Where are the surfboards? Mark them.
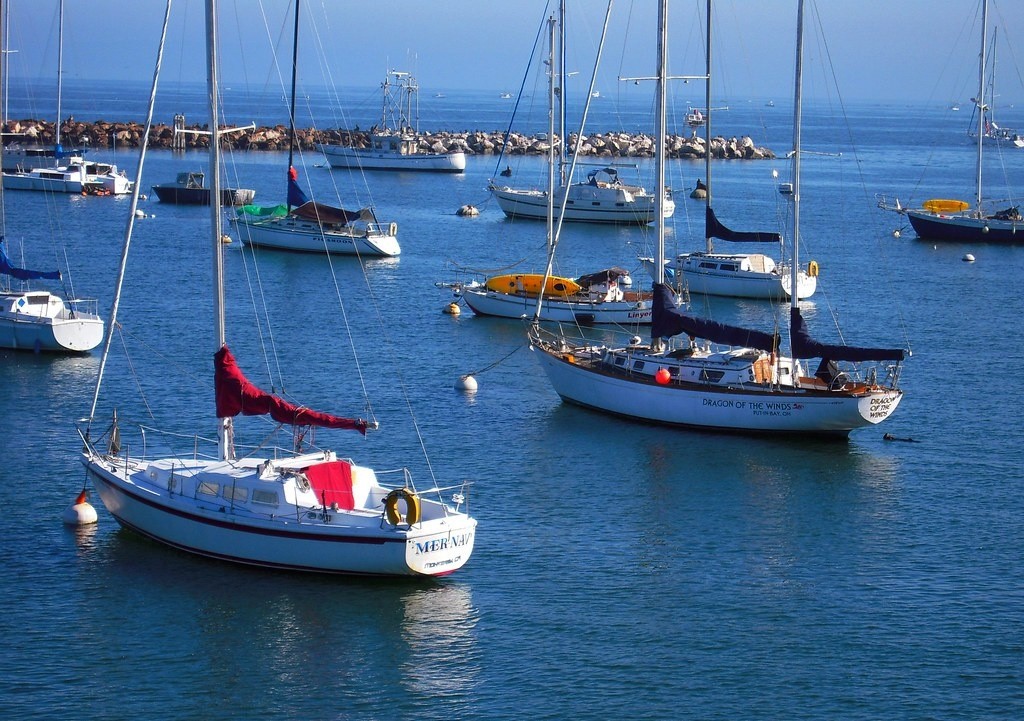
[922,200,969,213]
[486,274,581,298]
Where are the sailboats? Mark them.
[877,0,1024,244]
[524,0,916,433]
[486,0,677,226]
[636,0,821,300]
[433,0,692,326]
[0,0,107,355]
[0,0,135,196]
[964,22,1024,149]
[76,0,478,579]
[223,0,401,256]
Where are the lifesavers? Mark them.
[385,489,420,527]
[809,261,820,277]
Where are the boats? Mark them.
[592,91,601,99]
[764,100,776,107]
[951,106,959,111]
[434,93,447,99]
[779,180,793,194]
[312,50,469,174]
[150,171,258,204]
[684,109,706,126]
[498,92,513,100]
